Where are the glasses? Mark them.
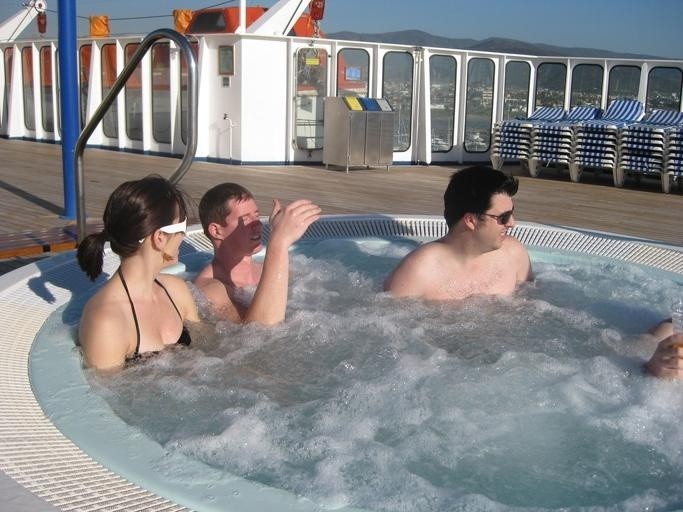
[476,206,515,226]
[159,216,188,236]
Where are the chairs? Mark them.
[491,98,683,195]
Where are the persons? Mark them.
[193,182,322,325]
[77,174,200,368]
[384,165,534,303]
[633,312,683,382]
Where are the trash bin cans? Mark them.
[322,97,397,174]
